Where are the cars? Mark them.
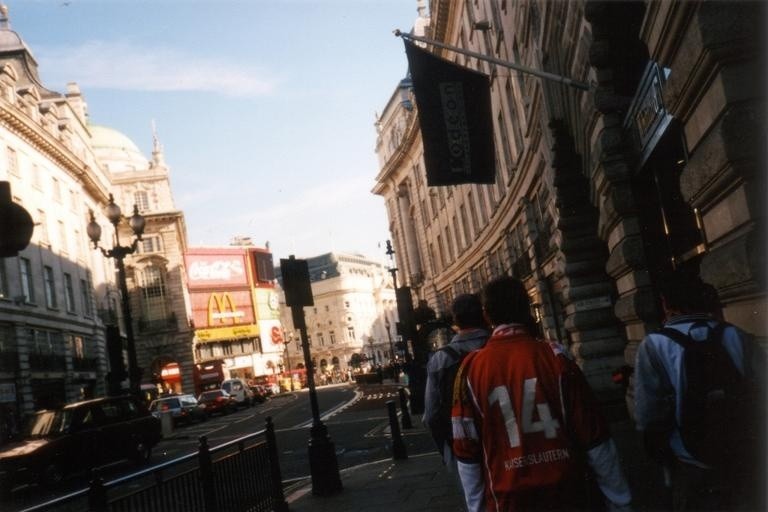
[148,373,280,426]
[1,397,162,487]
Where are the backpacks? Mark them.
[646,318,757,464]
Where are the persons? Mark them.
[632,271,766,512]
[420,292,493,495]
[448,275,636,512]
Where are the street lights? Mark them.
[86,194,145,391]
[367,335,378,365]
[383,309,394,358]
[282,326,293,391]
[385,240,399,290]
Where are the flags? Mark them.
[403,39,497,189]
